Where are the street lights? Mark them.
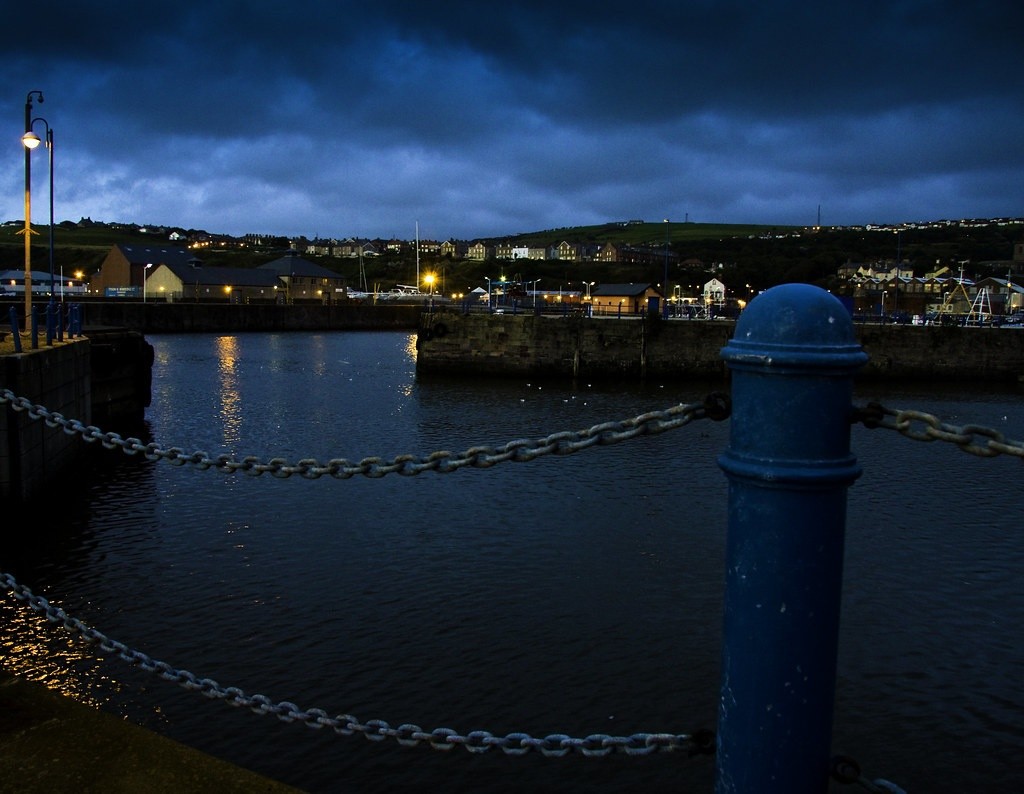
[745,283,751,306]
[582,281,590,298]
[588,282,596,313]
[25,89,46,333]
[143,263,153,302]
[426,274,435,295]
[484,276,492,311]
[23,116,55,306]
[662,218,671,297]
[532,278,542,308]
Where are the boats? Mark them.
[338,220,452,303]
